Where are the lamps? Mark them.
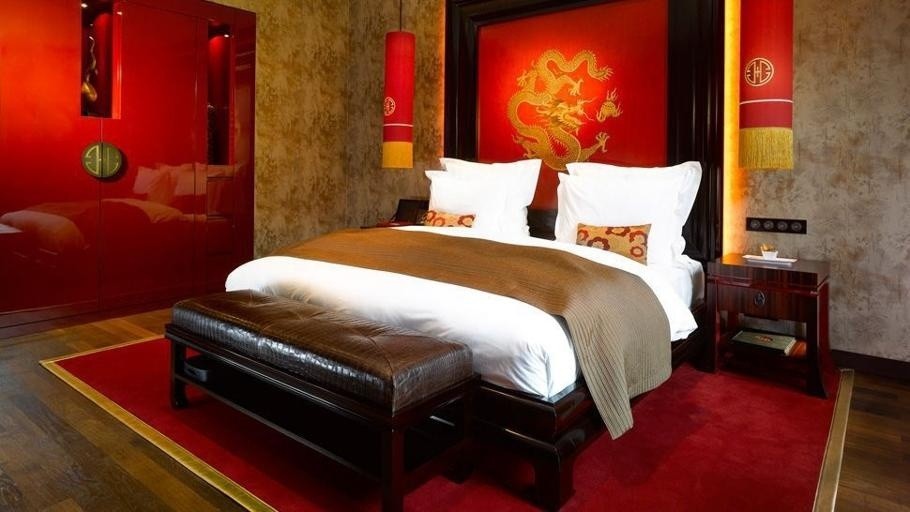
[738,1,796,173]
[379,0,417,172]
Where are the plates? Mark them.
[742,254,798,266]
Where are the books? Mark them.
[732,331,797,357]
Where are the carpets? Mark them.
[36,333,857,510]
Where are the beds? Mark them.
[224,198,705,510]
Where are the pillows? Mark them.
[420,157,704,266]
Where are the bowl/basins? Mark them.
[762,250,779,259]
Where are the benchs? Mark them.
[162,288,482,511]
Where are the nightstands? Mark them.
[702,254,833,398]
